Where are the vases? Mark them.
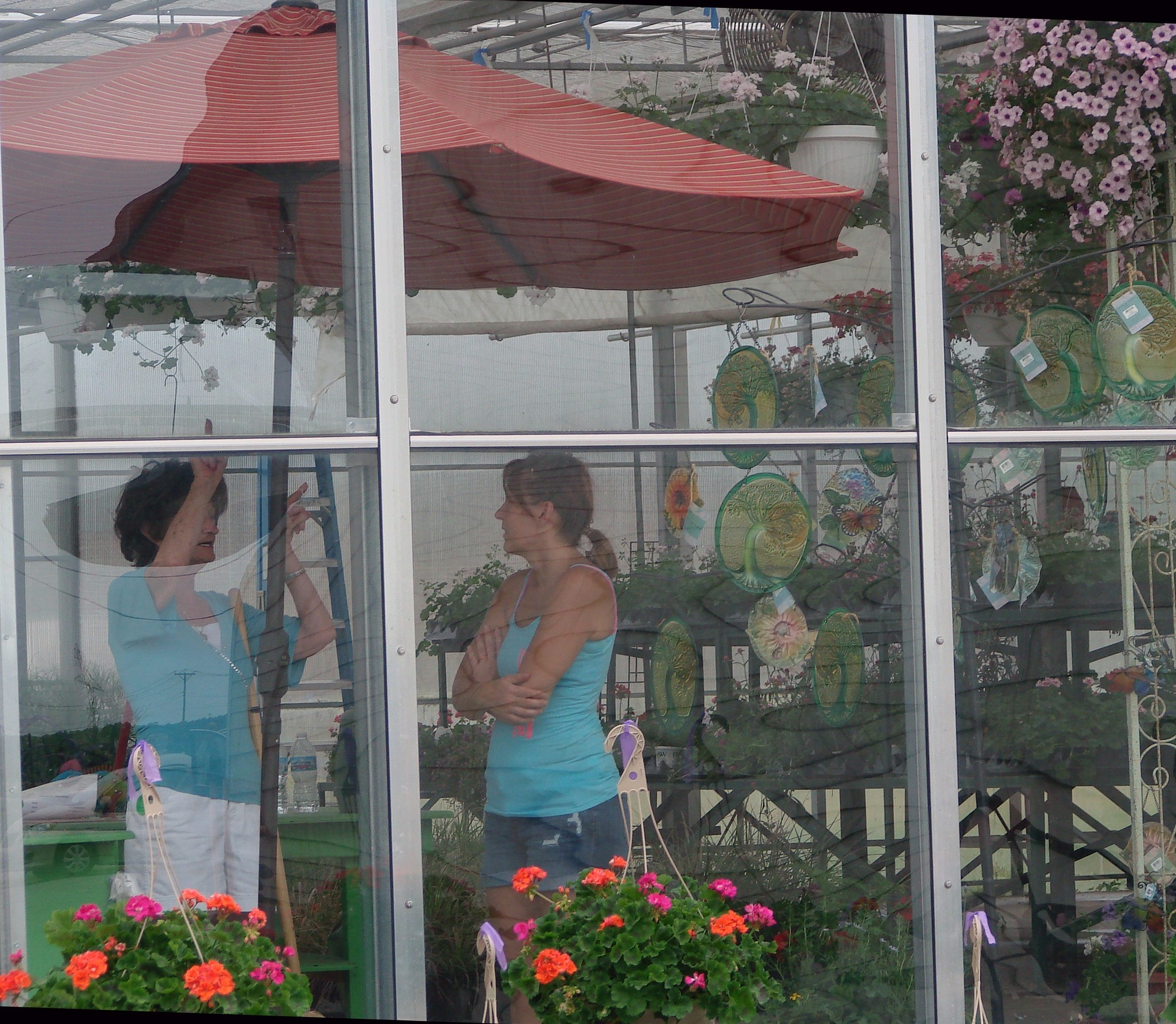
[30,287,110,345]
[961,303,1026,348]
[184,285,252,320]
[788,124,884,201]
[861,316,894,358]
[1125,969,1174,1020]
[108,283,176,333]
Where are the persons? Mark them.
[452,452,631,1022]
[104,454,337,910]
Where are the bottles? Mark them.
[291,732,320,813]
[277,744,288,813]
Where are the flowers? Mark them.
[281,843,500,1003]
[0,891,313,1018]
[572,19,1176,427]
[10,258,347,394]
[295,451,1176,1024]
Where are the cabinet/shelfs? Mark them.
[421,608,1176,995]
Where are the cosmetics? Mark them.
[0,0,868,947]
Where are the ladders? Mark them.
[256,452,354,937]
